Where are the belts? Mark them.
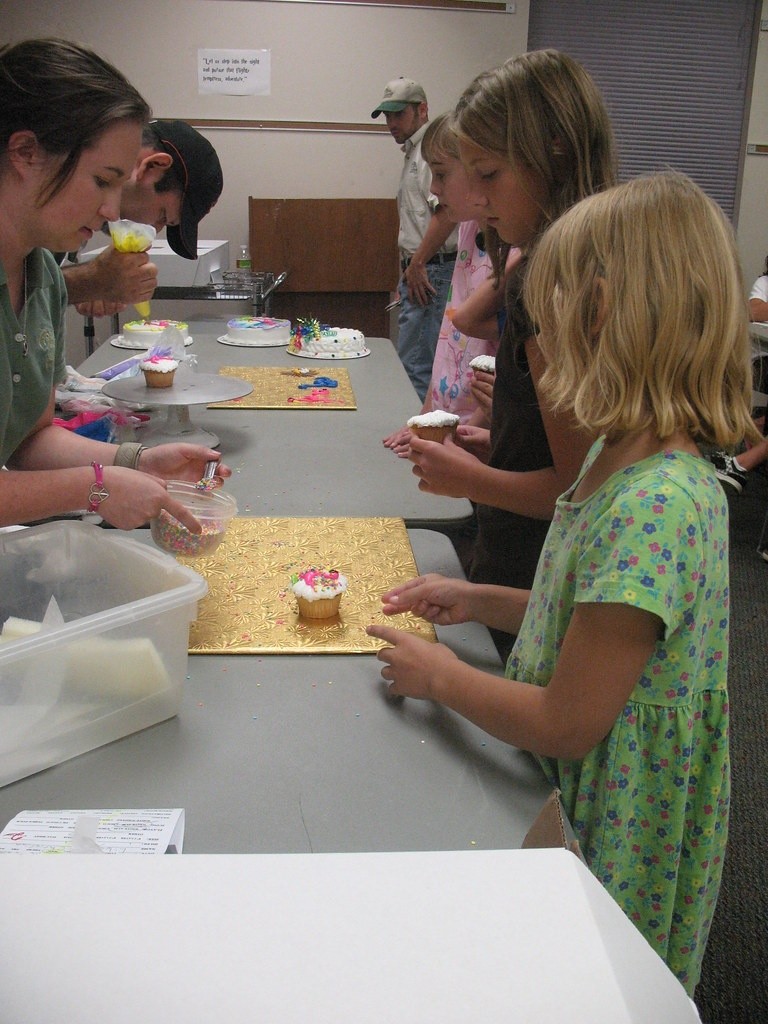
[401,252,459,272]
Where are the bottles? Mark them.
[235,245,253,290]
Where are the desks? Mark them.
[0,528,584,864]
[53,318,474,522]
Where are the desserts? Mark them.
[468,355,495,375]
[139,355,178,387]
[407,409,460,444]
[289,568,348,618]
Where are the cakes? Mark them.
[288,315,367,357]
[224,316,292,345]
[118,318,188,347]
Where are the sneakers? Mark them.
[702,449,748,496]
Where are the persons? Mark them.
[371,79,460,403]
[49,118,224,317]
[704,252,768,562]
[363,170,754,1003]
[409,47,617,662]
[0,36,233,533]
[381,107,526,463]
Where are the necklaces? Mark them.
[8,255,29,357]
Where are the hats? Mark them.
[149,118,222,260]
[371,77,426,119]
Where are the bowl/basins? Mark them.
[149,478,237,559]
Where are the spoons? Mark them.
[197,453,217,491]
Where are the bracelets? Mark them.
[87,460,109,513]
[113,441,148,470]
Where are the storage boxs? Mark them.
[0,521,206,792]
